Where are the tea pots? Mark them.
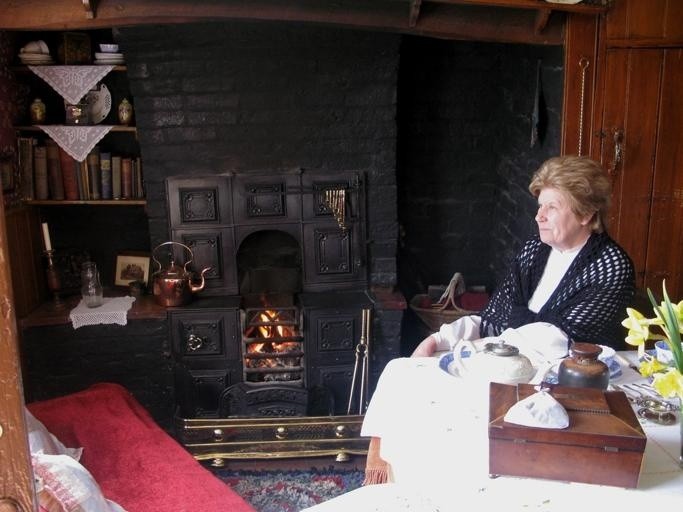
[148,241,212,305]
[453,338,554,389]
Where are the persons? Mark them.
[408,154,637,361]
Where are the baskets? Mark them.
[409,272,483,331]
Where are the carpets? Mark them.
[214,466,365,511]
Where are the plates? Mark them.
[63,84,111,125]
[438,349,467,378]
[637,348,657,376]
[92,53,124,65]
[18,53,51,64]
[604,359,621,375]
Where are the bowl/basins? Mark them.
[591,344,615,366]
[18,40,48,54]
[98,43,118,52]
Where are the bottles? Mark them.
[76,262,103,307]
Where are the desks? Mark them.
[359,345,683,510]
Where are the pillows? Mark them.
[21,405,129,512]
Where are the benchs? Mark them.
[16,382,256,512]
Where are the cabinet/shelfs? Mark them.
[561,0,683,349]
[1,30,168,325]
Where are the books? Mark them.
[16,138,146,201]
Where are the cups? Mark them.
[653,340,674,364]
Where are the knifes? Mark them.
[613,382,678,406]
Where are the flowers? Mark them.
[622,277,683,414]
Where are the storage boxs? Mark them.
[488,381,647,490]
